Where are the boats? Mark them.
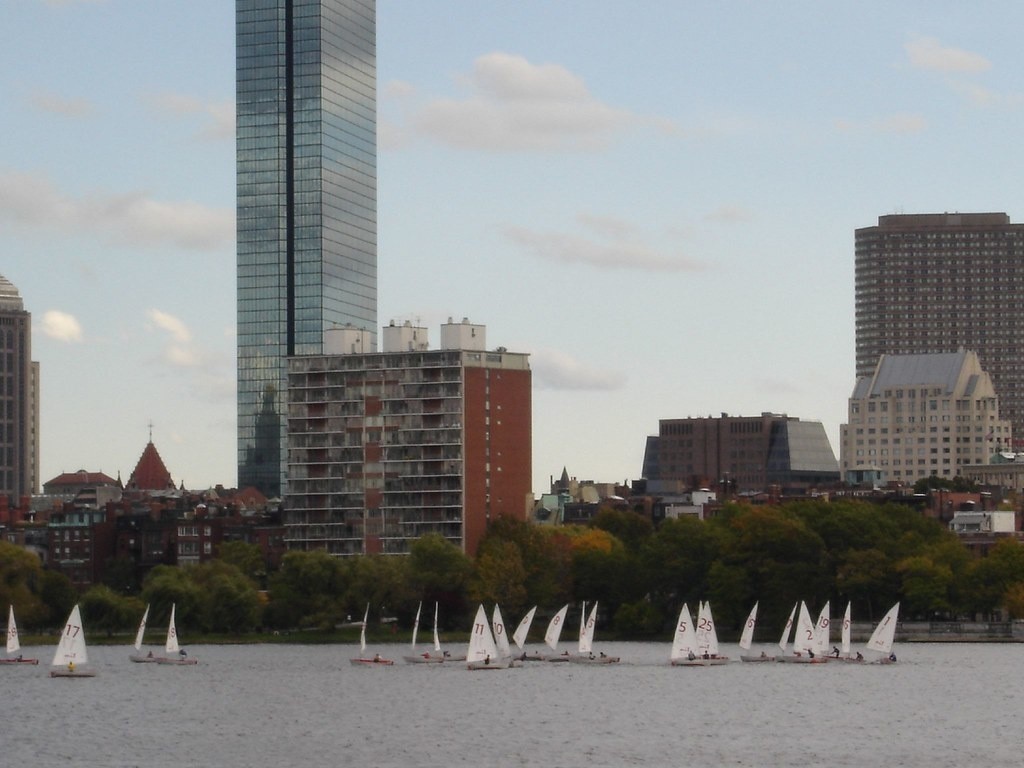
[0,605,39,666]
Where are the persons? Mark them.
[421,650,431,660]
[889,652,896,661]
[808,650,814,658]
[857,652,863,661]
[446,651,450,657]
[519,652,527,661]
[373,653,380,663]
[565,650,569,655]
[68,662,74,672]
[703,650,709,659]
[688,650,696,660]
[600,652,606,658]
[485,655,490,665]
[589,651,594,660]
[761,652,766,658]
[831,647,839,657]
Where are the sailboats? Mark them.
[466,603,523,670]
[544,601,621,667]
[350,602,394,666]
[739,600,900,664]
[513,605,545,661]
[670,600,729,666]
[130,604,198,664]
[403,601,467,663]
[48,605,96,678]
